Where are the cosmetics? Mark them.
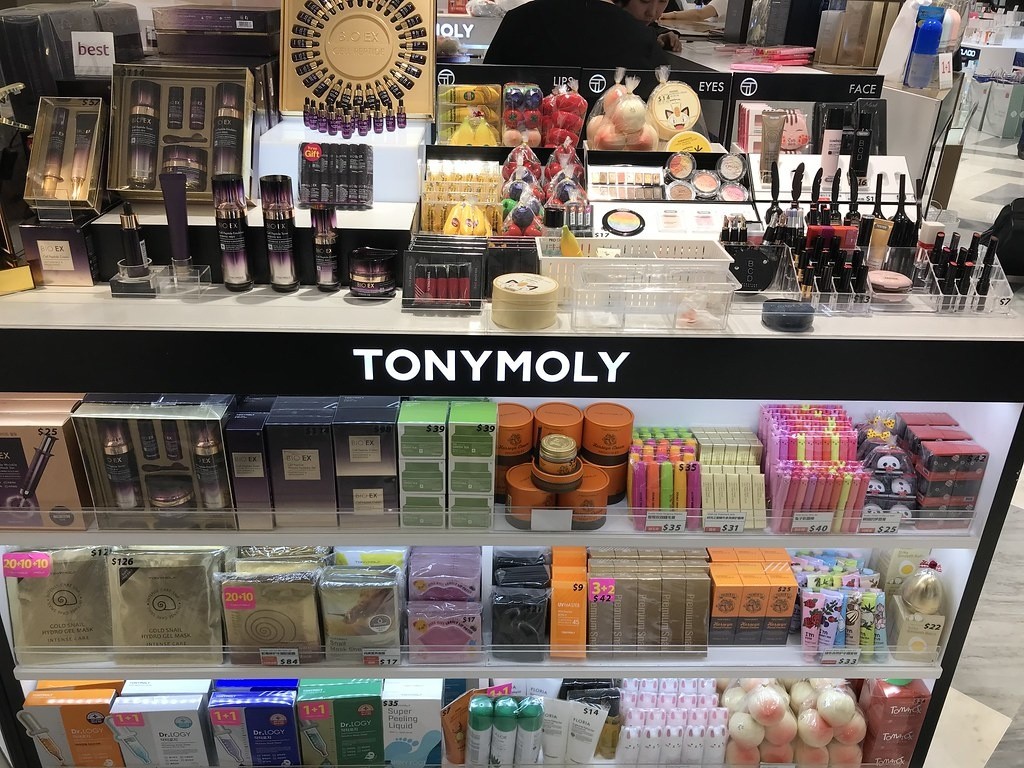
[0,0,999,768]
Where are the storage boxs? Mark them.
[0,391,798,768]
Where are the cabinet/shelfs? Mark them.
[659,41,835,155]
[0,281,1024,768]
[876,70,965,223]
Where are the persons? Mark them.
[656,0,728,22]
[618,0,682,54]
